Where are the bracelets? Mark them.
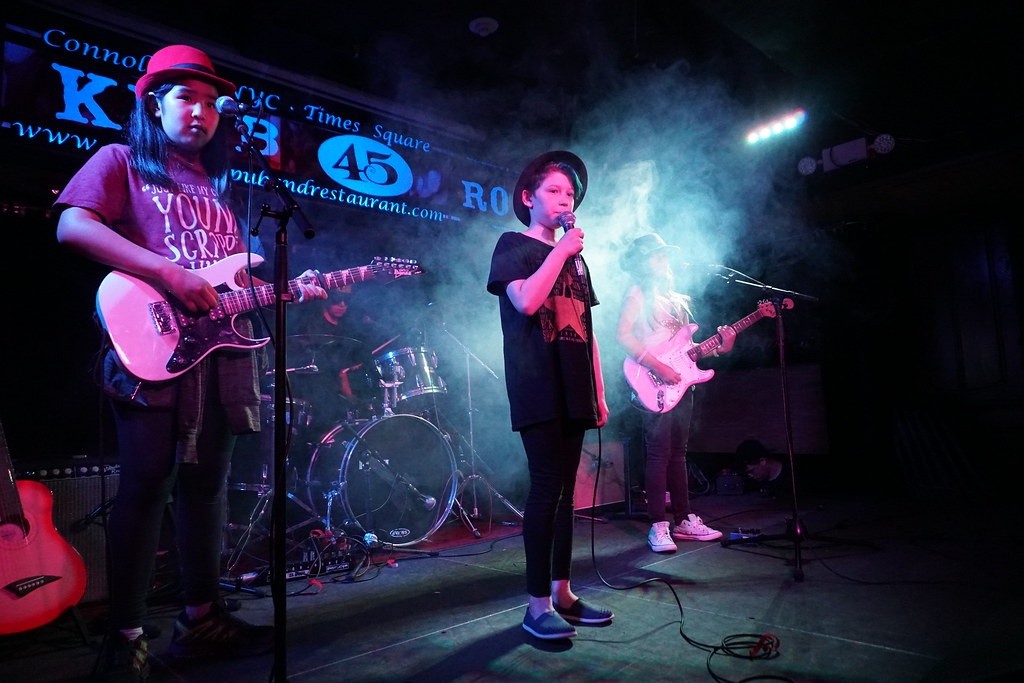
[635,350,649,364]
[712,349,720,357]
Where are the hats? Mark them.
[135,45,237,101]
[619,233,680,270]
[513,151,588,228]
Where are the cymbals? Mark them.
[287,334,372,368]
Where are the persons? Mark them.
[322,279,353,325]
[49,42,330,683]
[733,438,798,498]
[612,234,737,552]
[484,149,614,640]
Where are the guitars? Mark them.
[622,297,784,415]
[0,425,89,636]
[95,252,429,386]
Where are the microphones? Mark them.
[683,263,724,271]
[558,211,585,284]
[85,501,114,523]
[216,95,249,117]
[417,494,437,511]
[591,462,614,470]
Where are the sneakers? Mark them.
[522,607,575,639]
[553,598,612,623]
[94,623,163,683]
[673,514,723,541]
[168,597,276,666]
[648,520,677,552]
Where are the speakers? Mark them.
[10,455,120,604]
[572,433,628,516]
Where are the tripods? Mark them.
[224,345,440,583]
[711,270,874,583]
[455,381,524,520]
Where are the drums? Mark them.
[251,396,309,465]
[305,412,461,550]
[367,346,449,412]
